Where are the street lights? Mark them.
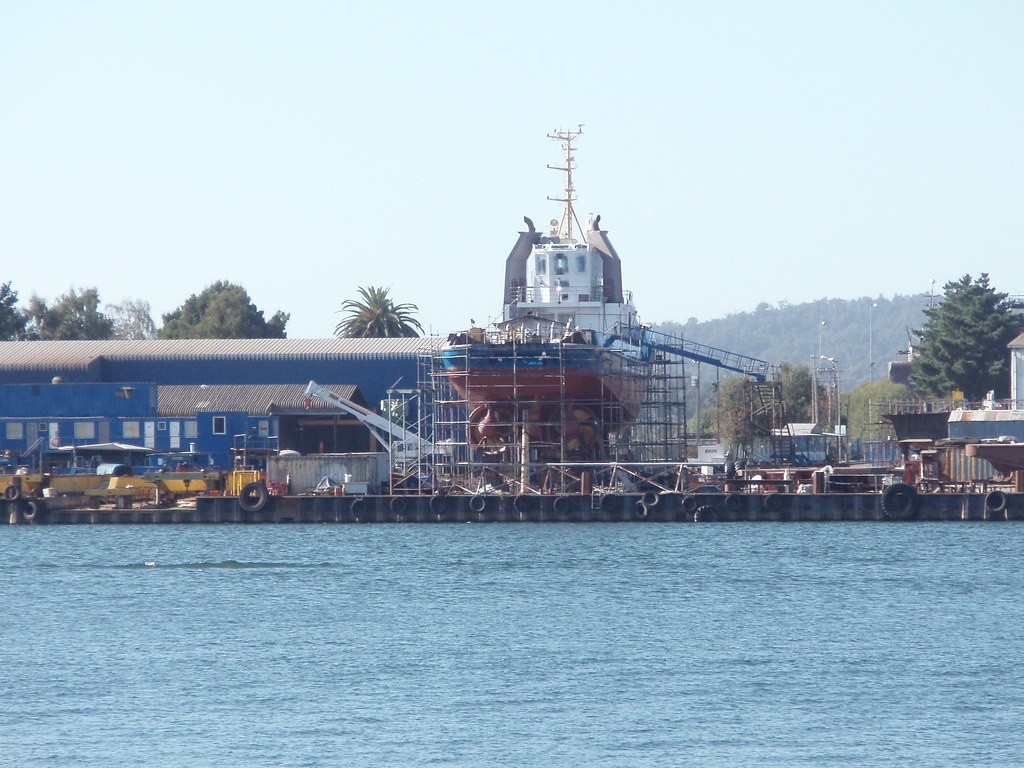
[819,355,841,460]
[818,319,827,369]
[868,302,878,459]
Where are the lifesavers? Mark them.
[555,497,572,514]
[513,494,535,511]
[763,494,785,513]
[725,493,741,511]
[389,496,407,515]
[469,496,487,512]
[985,489,1009,514]
[22,499,40,521]
[681,495,698,512]
[599,493,618,512]
[3,484,19,502]
[644,491,660,508]
[634,500,649,519]
[349,498,367,519]
[429,496,449,514]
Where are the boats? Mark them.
[438,121,772,490]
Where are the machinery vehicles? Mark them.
[302,380,455,495]
